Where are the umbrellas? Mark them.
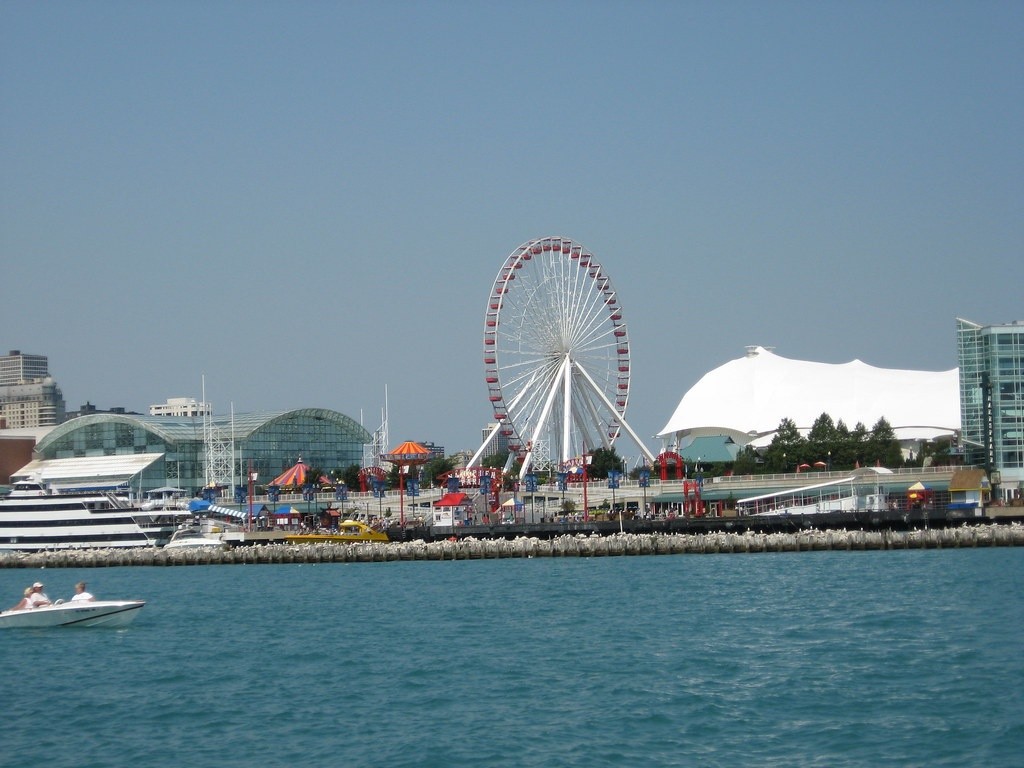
[502,498,524,521]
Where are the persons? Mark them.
[71,582,95,602]
[296,516,423,538]
[455,492,1021,523]
[11,582,54,611]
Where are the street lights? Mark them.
[909,445,912,465]
[828,450,833,470]
[783,453,786,473]
[621,455,626,480]
[698,457,702,474]
[625,461,628,479]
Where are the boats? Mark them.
[0,598,147,632]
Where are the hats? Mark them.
[33,583,43,589]
